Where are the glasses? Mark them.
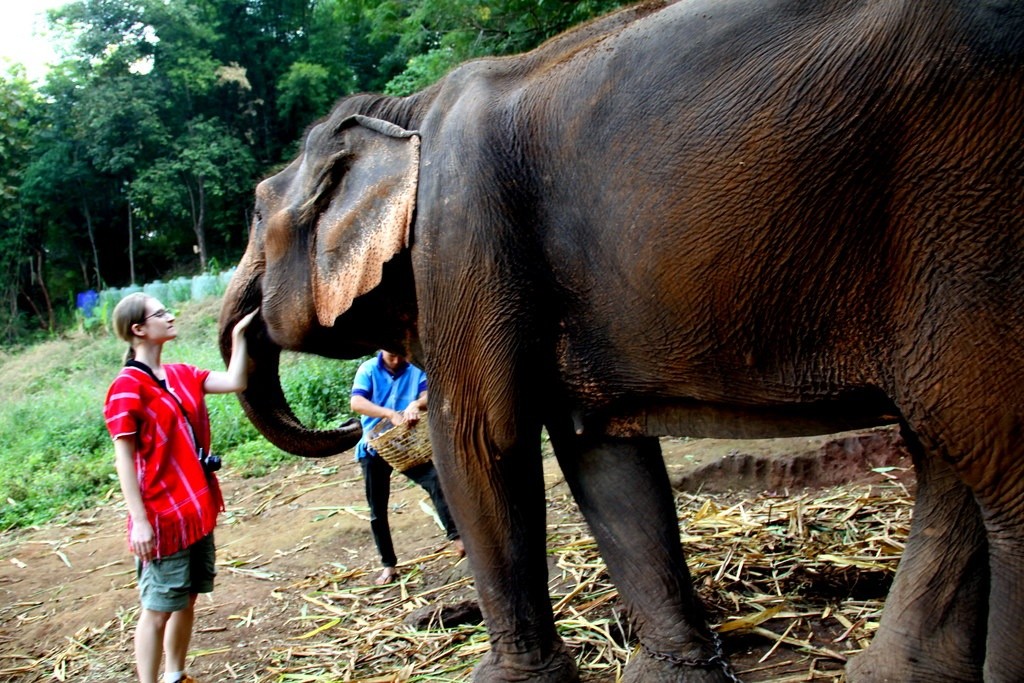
[144,308,171,320]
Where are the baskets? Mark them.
[366,410,431,472]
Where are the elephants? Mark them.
[217,0,1024,682]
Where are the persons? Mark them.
[350,349,468,589]
[101,291,260,683]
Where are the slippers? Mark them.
[381,572,399,585]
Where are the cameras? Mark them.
[197,448,222,480]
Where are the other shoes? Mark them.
[173,674,202,683]
